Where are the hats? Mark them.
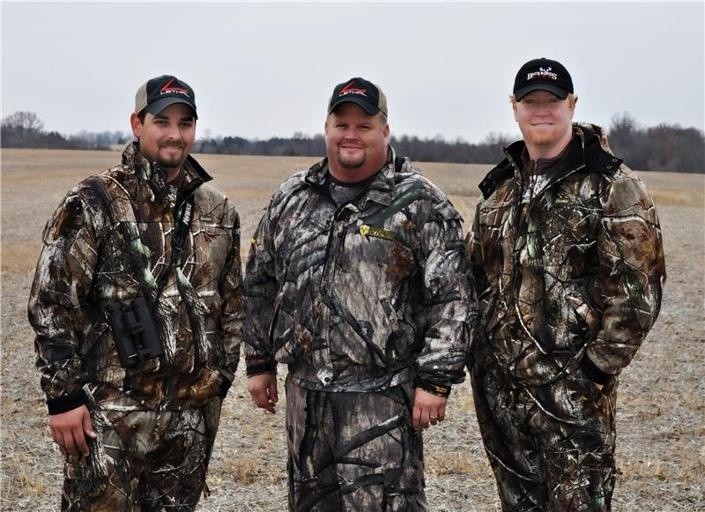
[512,58,574,102]
[134,72,198,120]
[327,76,388,118]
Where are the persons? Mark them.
[27,74,247,511]
[461,57,668,512]
[243,76,481,511]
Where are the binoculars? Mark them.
[107,297,163,368]
